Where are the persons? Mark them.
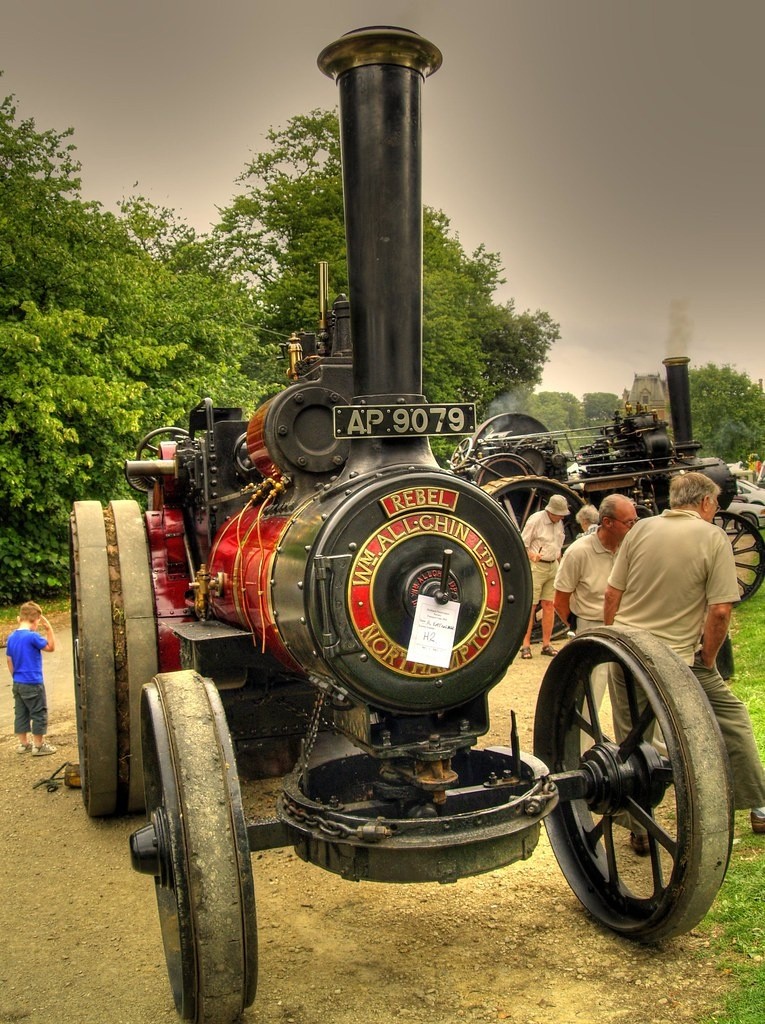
[566,451,590,491]
[6,601,57,756]
[520,493,668,757]
[604,472,765,855]
[747,453,765,484]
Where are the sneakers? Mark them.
[32,744,57,755]
[18,742,33,753]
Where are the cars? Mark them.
[728,463,765,505]
[712,502,764,533]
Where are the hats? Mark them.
[545,495,571,515]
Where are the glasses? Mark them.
[601,515,641,528]
[713,506,721,513]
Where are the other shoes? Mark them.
[750,812,765,833]
[630,831,658,857]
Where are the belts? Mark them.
[539,559,556,563]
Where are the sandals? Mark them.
[520,648,533,659]
[541,644,559,656]
